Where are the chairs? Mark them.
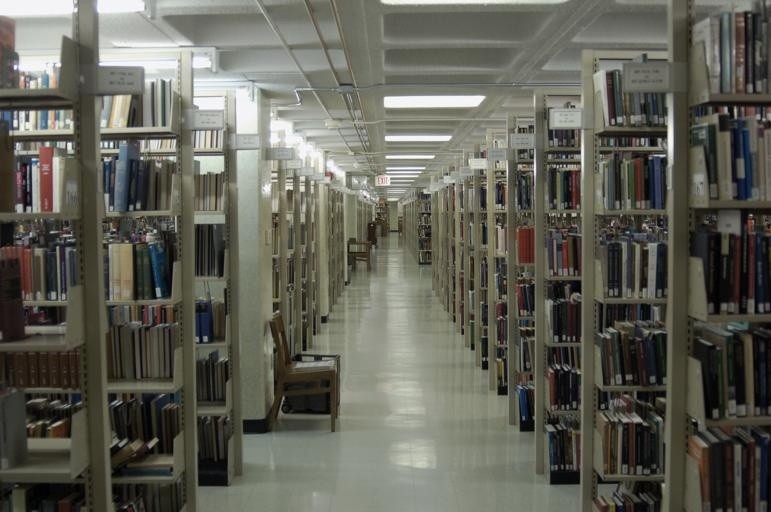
[265,308,343,435]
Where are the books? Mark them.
[686,1,770,511]
[515,125,534,422]
[271,182,317,356]
[544,101,582,471]
[98,78,187,510]
[1,16,85,511]
[593,52,666,511]
[193,130,229,464]
[494,140,508,386]
[419,191,431,262]
[446,150,488,369]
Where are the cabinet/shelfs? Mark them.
[403,0,771,511]
[1,0,373,512]
